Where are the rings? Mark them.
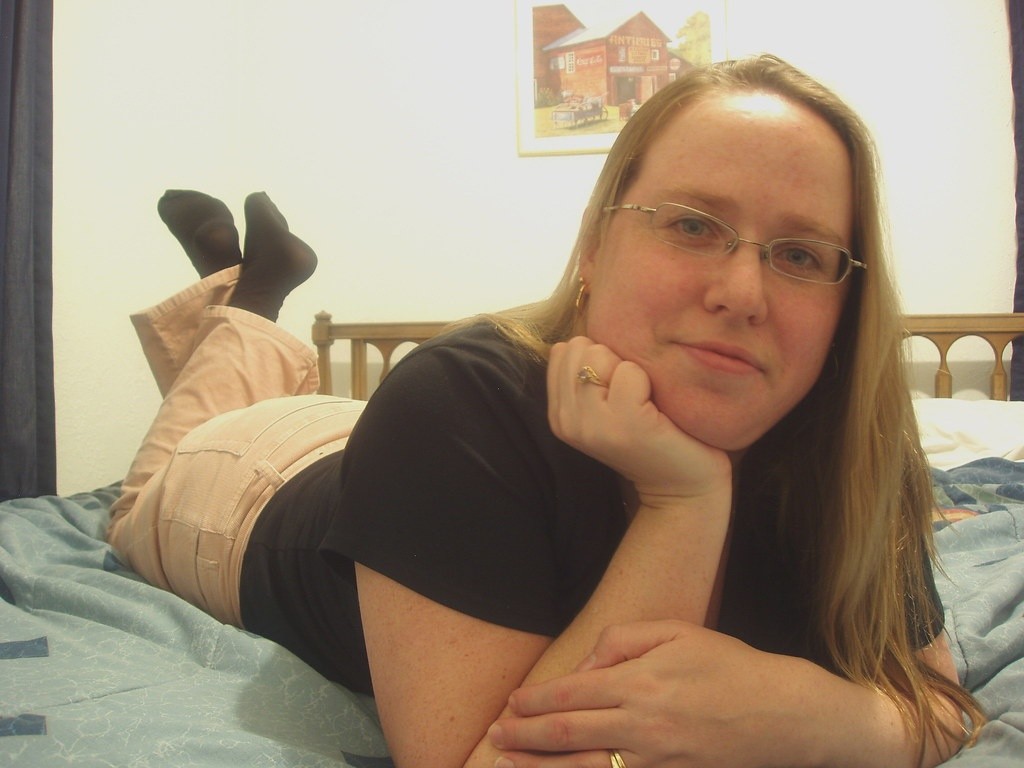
[609,750,626,768]
[575,365,611,388]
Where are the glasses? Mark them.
[602,202,868,286]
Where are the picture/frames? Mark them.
[513,0,730,158]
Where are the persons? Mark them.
[102,60,983,768]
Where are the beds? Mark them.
[0,312,1024,767]
